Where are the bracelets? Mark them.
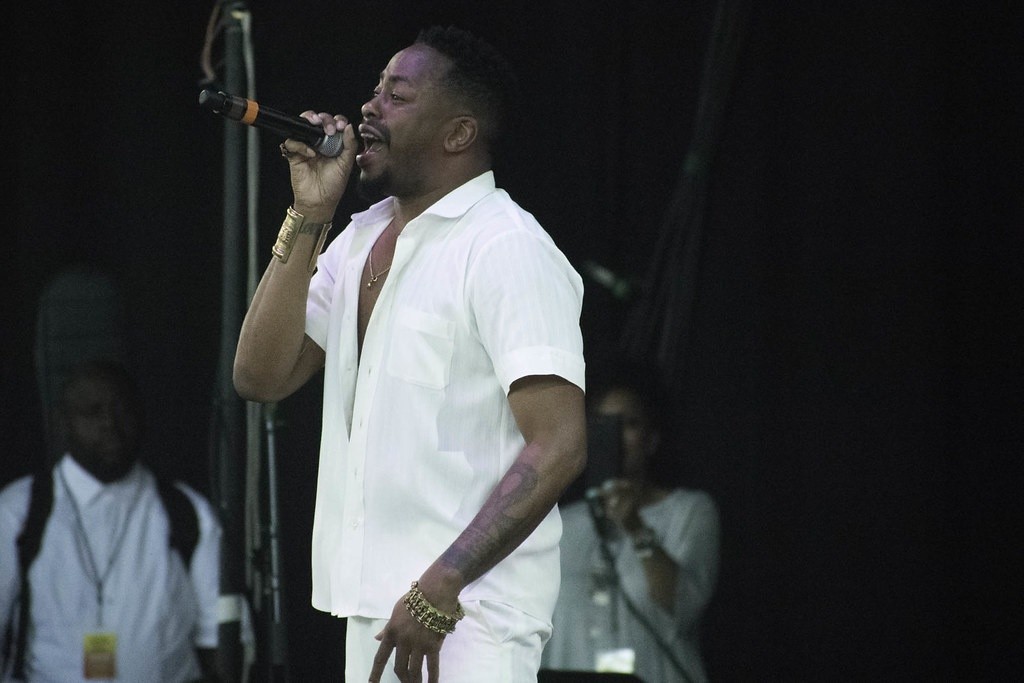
[271,205,332,273]
[405,582,466,634]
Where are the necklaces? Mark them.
[367,252,391,289]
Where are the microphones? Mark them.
[586,485,609,537]
[199,90,344,158]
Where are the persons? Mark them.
[231,27,586,682]
[0,359,206,683]
[541,382,722,683]
